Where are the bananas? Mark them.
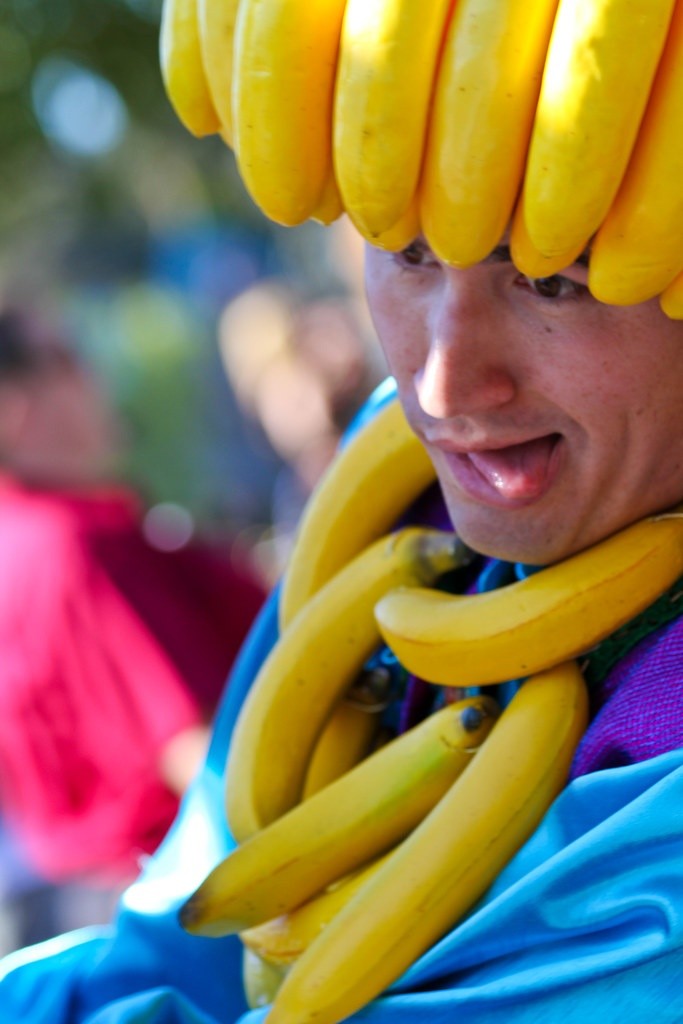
[178,396,683,1024]
[160,0,683,323]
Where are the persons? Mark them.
[2,276,276,939]
[223,285,385,535]
[0,1,682,1024]
